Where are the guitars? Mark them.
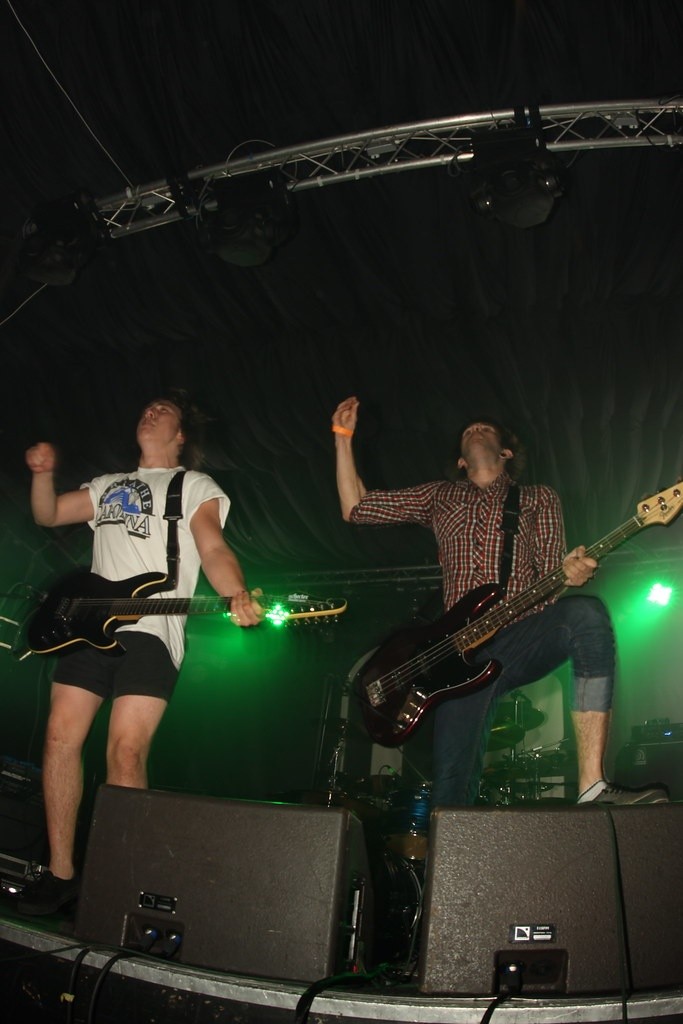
[21,568,348,658]
[356,473,683,749]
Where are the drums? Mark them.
[367,849,424,971]
[378,786,432,860]
[496,754,556,799]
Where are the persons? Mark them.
[330,393,670,807]
[17,387,266,916]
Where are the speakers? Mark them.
[73,783,375,984]
[418,806,683,996]
[623,740,683,803]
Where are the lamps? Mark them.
[12,188,112,287]
[469,125,564,229]
[194,179,297,267]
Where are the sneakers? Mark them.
[402,960,419,977]
[17,870,80,915]
[593,784,669,804]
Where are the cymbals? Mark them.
[265,788,383,824]
[485,719,526,753]
[494,701,546,731]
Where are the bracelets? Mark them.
[332,424,353,437]
